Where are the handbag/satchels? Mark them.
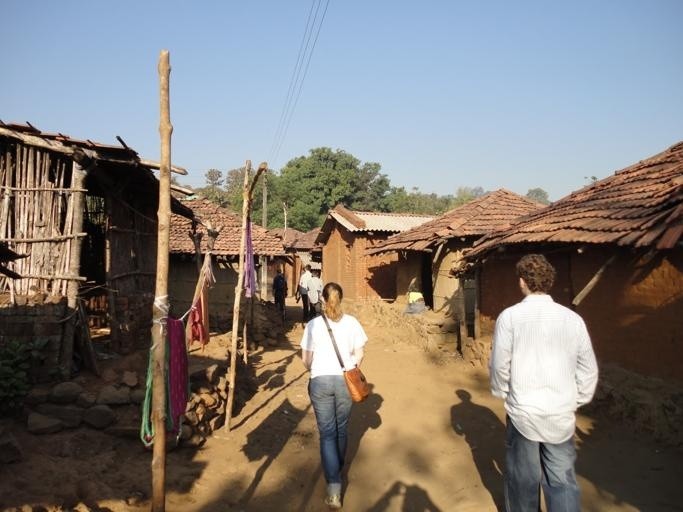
[342,367,370,403]
[306,289,320,305]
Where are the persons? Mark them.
[299,281,368,507]
[295,264,313,324]
[294,266,306,300]
[488,253,600,512]
[271,268,287,320]
[306,268,326,321]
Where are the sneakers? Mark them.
[324,496,342,509]
